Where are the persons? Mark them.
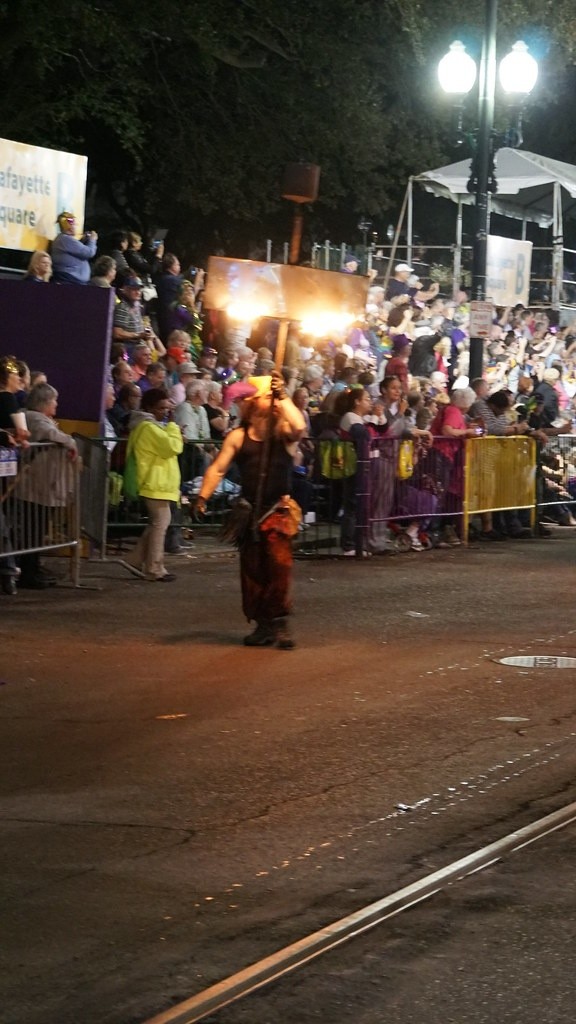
[23,211,576,557]
[117,388,183,582]
[0,353,78,586]
[188,369,306,649]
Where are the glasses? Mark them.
[58,215,74,226]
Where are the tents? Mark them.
[407,146,576,301]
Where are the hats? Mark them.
[124,275,144,287]
[201,347,219,355]
[177,362,201,374]
[391,334,413,348]
[395,264,415,272]
[344,254,361,263]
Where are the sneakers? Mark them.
[401,529,426,552]
[428,530,448,547]
[443,525,461,545]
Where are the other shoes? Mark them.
[364,538,406,554]
[340,542,371,557]
[273,620,297,650]
[244,622,274,646]
[480,528,506,541]
[558,512,576,526]
[290,546,316,560]
[121,559,143,577]
[164,547,188,556]
[165,533,196,549]
[16,567,57,585]
[143,572,178,582]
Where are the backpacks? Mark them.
[123,423,156,502]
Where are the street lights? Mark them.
[437,39,538,388]
